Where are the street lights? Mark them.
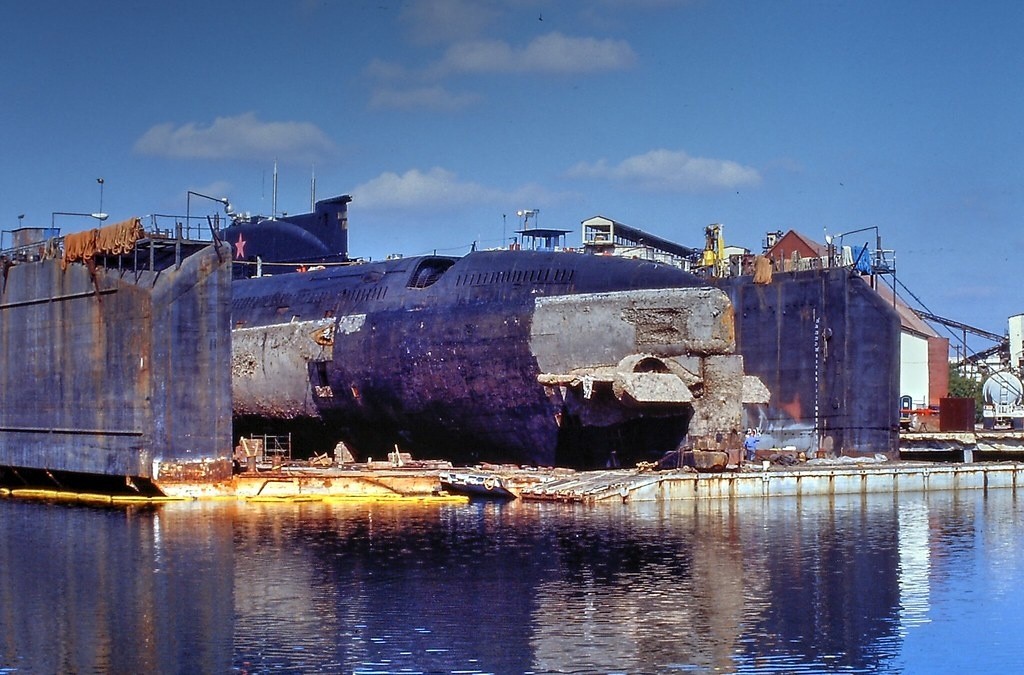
[185,190,234,241]
[823,226,880,273]
[52,211,108,229]
[17,214,25,228]
[95,178,105,231]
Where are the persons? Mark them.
[745,433,760,461]
